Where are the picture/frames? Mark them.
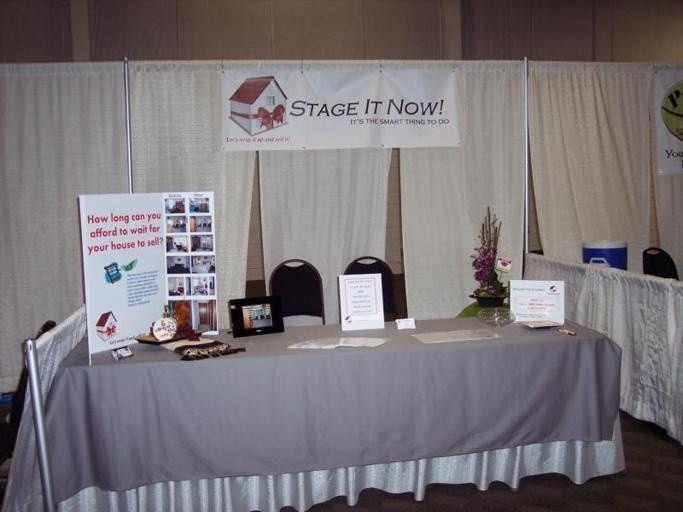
[470,295,509,307]
[229,296,285,338]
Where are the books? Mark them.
[286,334,391,349]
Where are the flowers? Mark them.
[470,206,511,297]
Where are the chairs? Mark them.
[643,246,680,281]
[343,255,396,321]
[269,259,326,326]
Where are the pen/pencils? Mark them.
[557,328,576,335]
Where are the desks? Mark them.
[57,311,606,512]
